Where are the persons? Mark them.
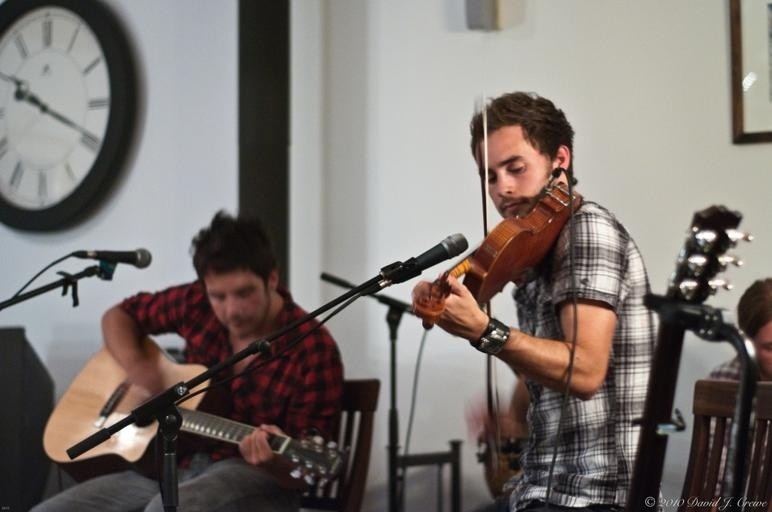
[701,278,772,511]
[464,373,532,512]
[27,208,342,511]
[435,90,661,512]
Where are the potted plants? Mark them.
[729,0,772,144]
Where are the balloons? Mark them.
[0,0,139,233]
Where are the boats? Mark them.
[466,0,527,31]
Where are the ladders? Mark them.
[414,181,583,330]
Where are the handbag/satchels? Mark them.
[470,317,510,356]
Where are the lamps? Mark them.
[676,378,772,512]
[299,379,380,512]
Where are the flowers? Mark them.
[72,248,153,269]
[360,232,469,297]
[515,168,562,219]
